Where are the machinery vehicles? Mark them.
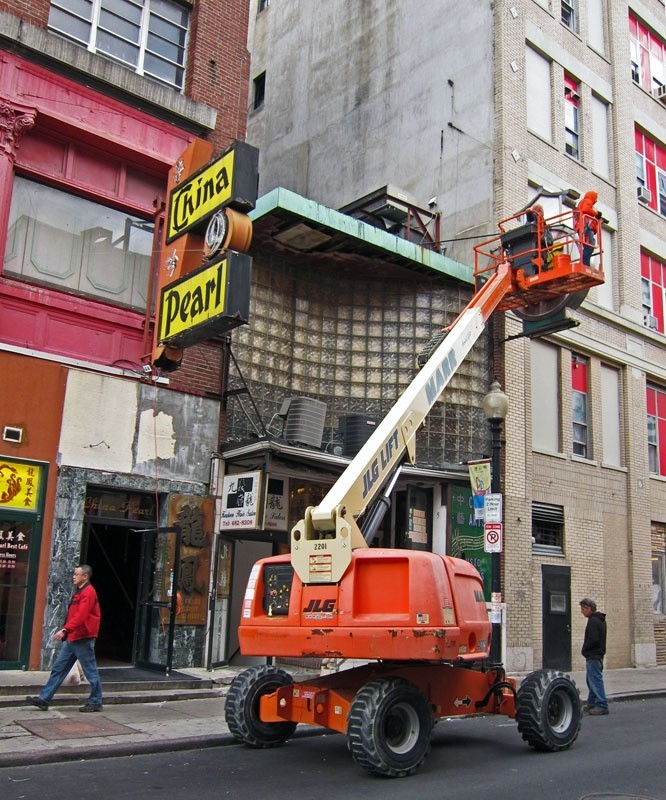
[222,190,607,779]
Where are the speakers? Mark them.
[339,414,381,460]
[279,396,327,449]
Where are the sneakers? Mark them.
[79,704,103,712]
[26,696,48,711]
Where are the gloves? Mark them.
[601,218,609,224]
[597,212,602,219]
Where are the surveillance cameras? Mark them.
[427,196,438,209]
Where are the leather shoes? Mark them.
[583,706,593,712]
[589,708,609,715]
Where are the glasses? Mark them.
[73,573,83,576]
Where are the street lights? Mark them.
[479,381,507,670]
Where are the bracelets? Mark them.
[62,629,67,634]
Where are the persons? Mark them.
[527,205,552,271]
[579,598,610,715]
[576,191,602,266]
[28,563,104,712]
